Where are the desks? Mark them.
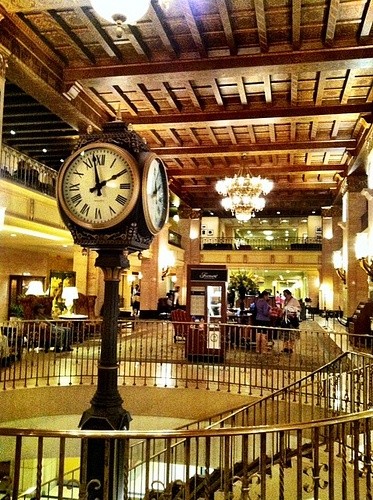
[59,315,88,344]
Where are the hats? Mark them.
[34,304,43,309]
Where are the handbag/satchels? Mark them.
[251,302,257,317]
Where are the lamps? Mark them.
[333,251,346,284]
[355,233,373,282]
[214,155,274,221]
[89,0,151,38]
[61,287,79,316]
[25,281,43,295]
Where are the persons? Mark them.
[33,303,74,352]
[172,285,181,310]
[254,290,273,355]
[131,284,141,319]
[276,290,302,353]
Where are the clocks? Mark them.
[58,141,141,231]
[141,152,169,235]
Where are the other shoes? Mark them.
[281,348,293,354]
[53,348,61,353]
[62,347,73,351]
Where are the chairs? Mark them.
[19,294,64,351]
[171,309,187,343]
[74,293,135,337]
